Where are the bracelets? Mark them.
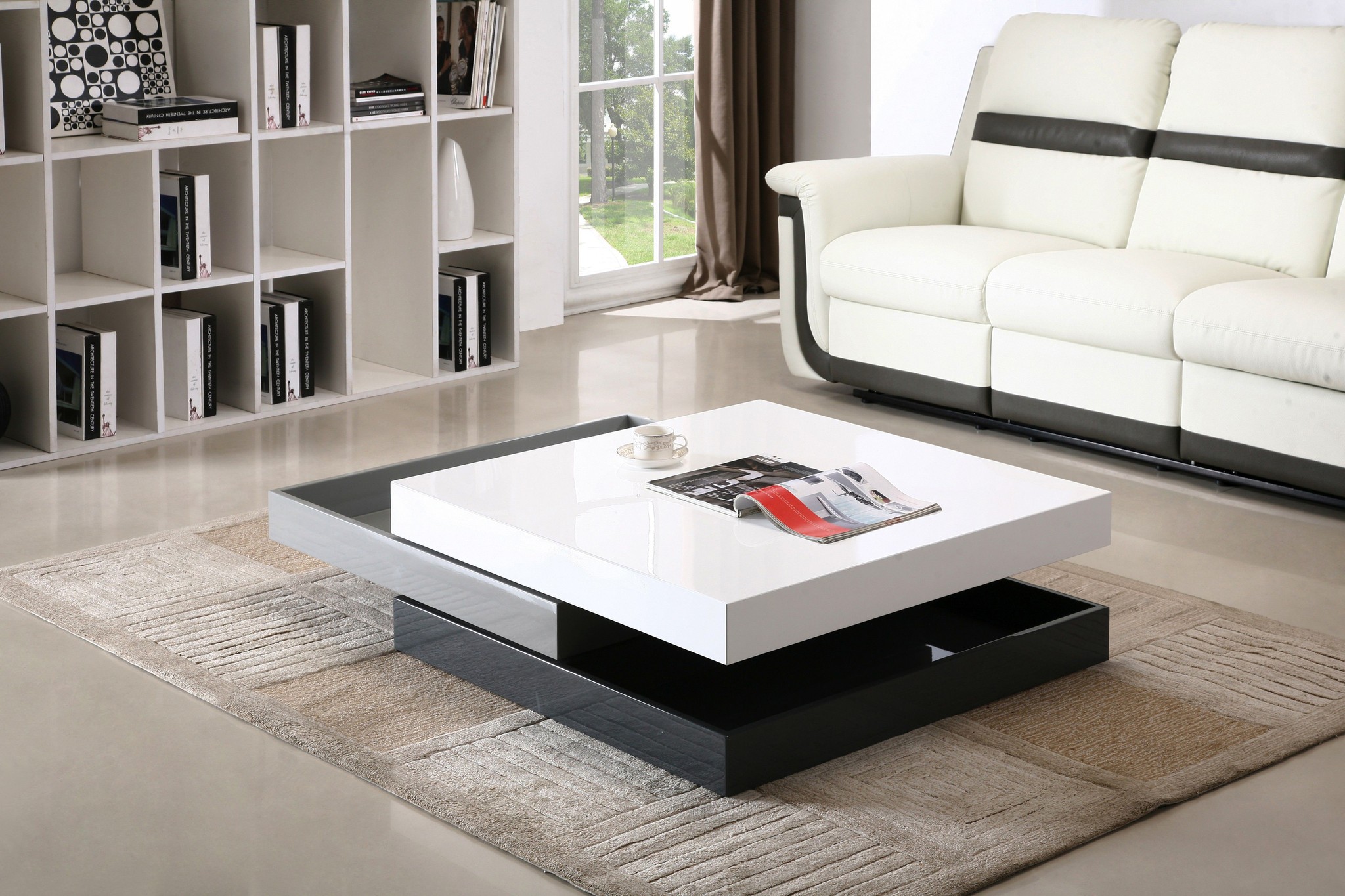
[438,72,442,76]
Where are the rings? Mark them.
[450,59,451,60]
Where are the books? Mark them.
[261,290,315,405]
[438,266,491,372]
[350,73,426,122]
[159,171,212,281]
[56,321,116,441]
[646,454,942,544]
[436,0,506,109]
[101,91,239,142]
[256,22,310,129]
[162,307,217,421]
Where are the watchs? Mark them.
[449,58,468,95]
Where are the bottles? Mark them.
[438,136,474,241]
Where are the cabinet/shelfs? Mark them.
[0,0,523,469]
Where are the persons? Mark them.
[437,16,452,95]
[457,5,477,96]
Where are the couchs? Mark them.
[764,14,1345,513]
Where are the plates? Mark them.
[616,442,689,469]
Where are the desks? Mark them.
[263,405,1115,799]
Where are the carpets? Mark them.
[0,499,1344,896]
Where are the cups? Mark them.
[633,426,688,460]
